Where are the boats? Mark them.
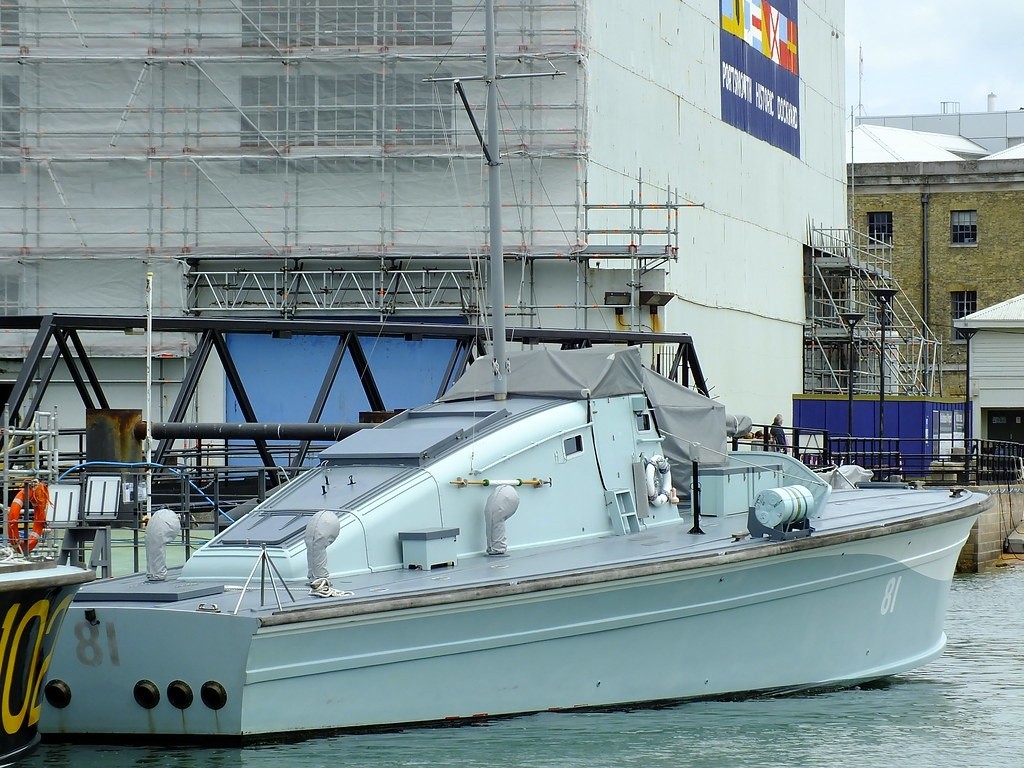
[0,390,97,768]
[38,0,997,750]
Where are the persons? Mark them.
[770,413,788,454]
[741,431,764,439]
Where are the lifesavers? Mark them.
[7,487,46,554]
